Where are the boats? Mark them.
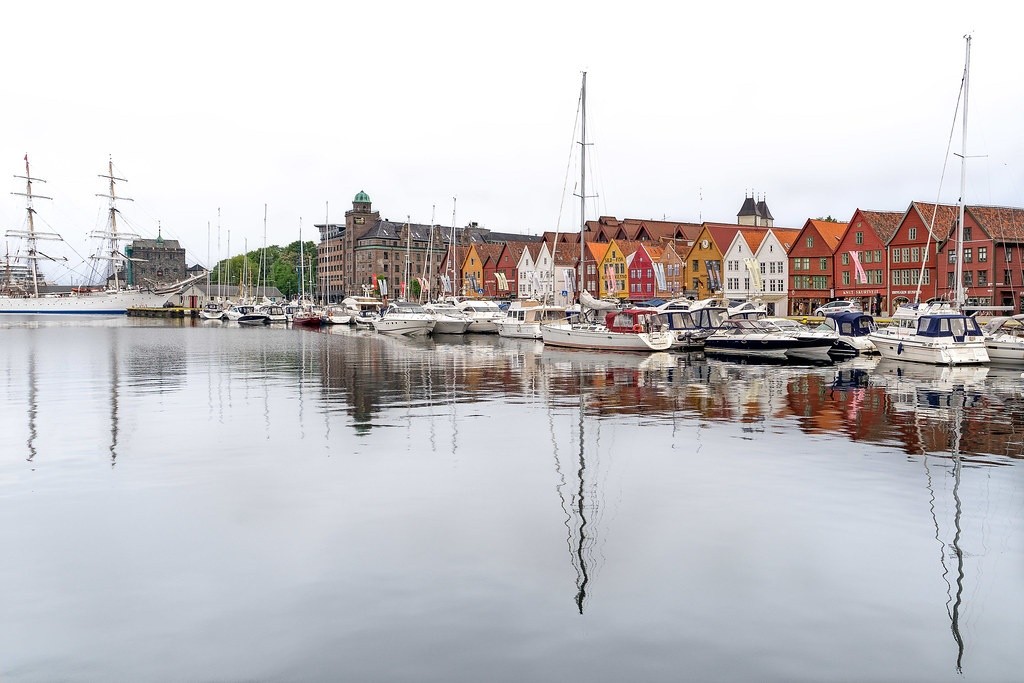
[199,31,879,360]
[983,313,1024,367]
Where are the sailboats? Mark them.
[538,70,675,352]
[866,32,990,365]
[0,150,213,317]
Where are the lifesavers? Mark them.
[633,324,642,332]
[328,312,332,316]
[578,313,587,323]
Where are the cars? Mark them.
[813,300,864,318]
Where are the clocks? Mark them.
[702,240,708,249]
